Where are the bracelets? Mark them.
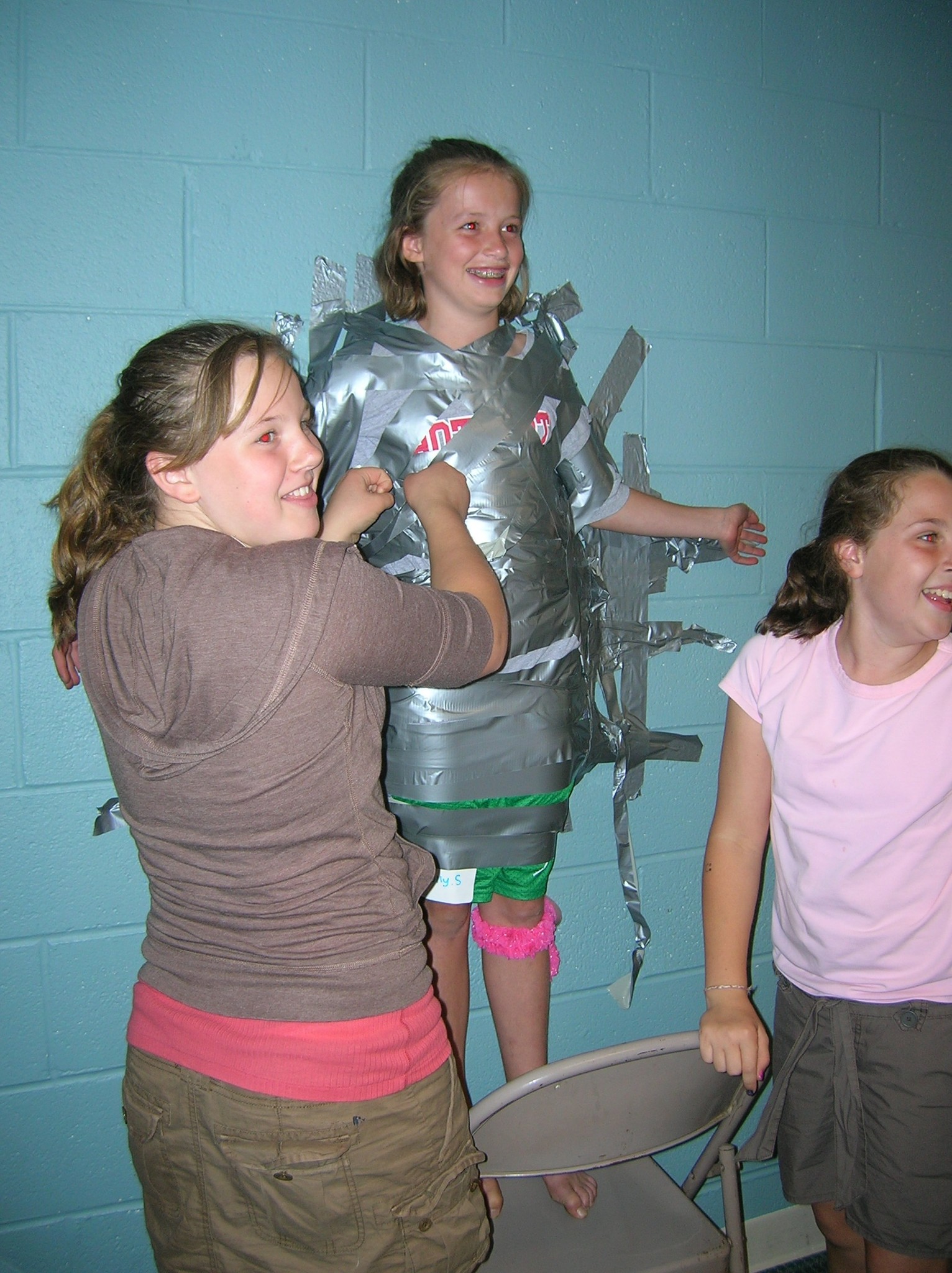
[704,983,754,997]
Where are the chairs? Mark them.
[467,1030,767,1272]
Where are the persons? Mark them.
[46,135,769,1226]
[40,321,504,1273]
[699,449,951,1273]
